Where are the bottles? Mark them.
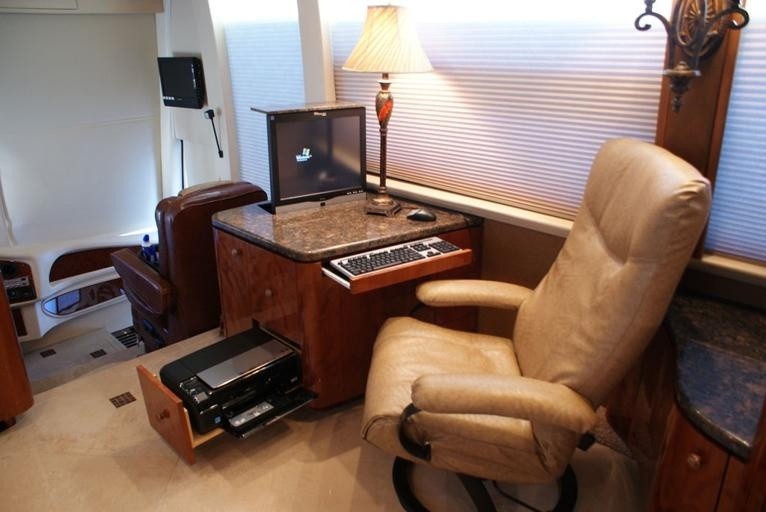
[142,235,157,262]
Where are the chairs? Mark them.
[359,136,712,512]
[109,180,267,354]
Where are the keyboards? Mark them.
[329,235,462,280]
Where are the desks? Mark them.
[135,192,483,466]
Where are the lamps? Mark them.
[341,4,435,218]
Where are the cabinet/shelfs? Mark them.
[605,323,766,512]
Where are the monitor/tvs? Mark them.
[257,105,367,215]
[157,57,205,109]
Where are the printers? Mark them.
[160,328,319,438]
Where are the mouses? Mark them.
[407,209,436,221]
